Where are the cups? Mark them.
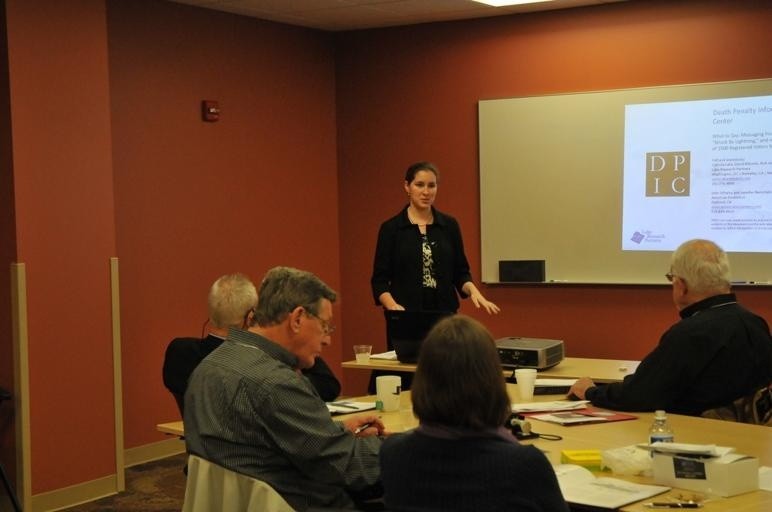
[353,344,373,362]
[516,368,537,400]
[376,374,401,412]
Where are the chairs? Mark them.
[181,454,294,512]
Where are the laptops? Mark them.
[384,310,456,363]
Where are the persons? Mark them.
[370,160,501,364]
[565,239,771,427]
[182,263,392,512]
[160,271,343,474]
[379,312,570,512]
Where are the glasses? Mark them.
[296,304,336,336]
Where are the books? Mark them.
[554,463,672,511]
[520,413,639,427]
[327,401,378,417]
[511,400,592,412]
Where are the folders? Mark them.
[516,404,639,425]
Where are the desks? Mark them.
[159,380,772,512]
[342,348,642,384]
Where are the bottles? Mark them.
[649,410,673,457]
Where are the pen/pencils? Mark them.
[331,403,359,409]
[354,416,382,434]
[643,501,704,509]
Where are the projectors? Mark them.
[495,336,564,369]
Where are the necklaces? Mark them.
[410,210,433,227]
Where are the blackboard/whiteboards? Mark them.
[477,77,772,288]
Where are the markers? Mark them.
[749,281,769,285]
